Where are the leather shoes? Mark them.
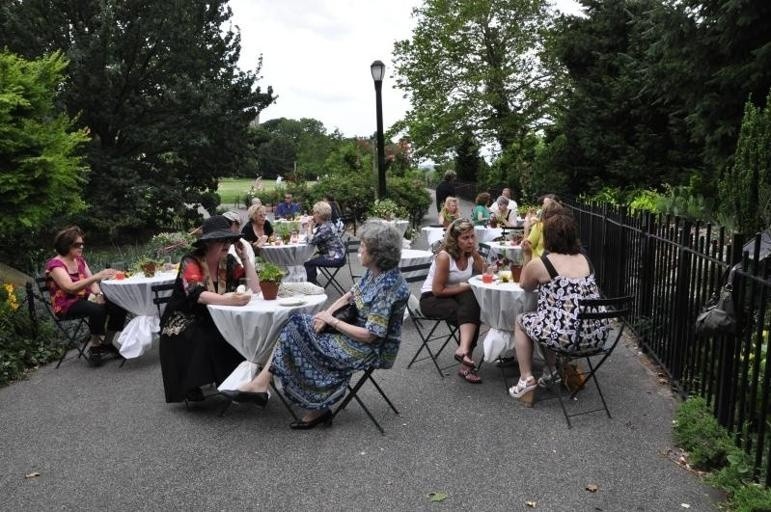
[186,388,205,402]
[89,346,112,361]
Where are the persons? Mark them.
[322,193,340,222]
[492,188,518,210]
[222,211,256,274]
[470,193,492,226]
[304,202,347,283]
[221,222,410,429]
[439,197,460,226]
[275,194,300,219]
[544,195,561,203]
[160,215,261,403]
[523,202,564,258]
[436,170,457,212]
[47,227,127,359]
[509,216,611,405]
[494,196,517,228]
[420,217,485,382]
[241,205,274,243]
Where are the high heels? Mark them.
[290,410,333,430]
[220,389,268,409]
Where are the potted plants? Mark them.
[252,261,283,300]
[277,225,291,244]
[134,254,163,278]
[510,250,524,283]
[516,205,528,219]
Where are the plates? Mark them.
[280,298,305,306]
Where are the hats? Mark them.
[191,215,248,247]
[222,211,243,225]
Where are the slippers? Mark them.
[454,353,476,368]
[458,369,481,383]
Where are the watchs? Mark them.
[95,291,103,297]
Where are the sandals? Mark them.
[537,370,561,393]
[495,356,514,366]
[508,376,537,408]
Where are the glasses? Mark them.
[217,237,237,245]
[73,242,86,248]
[284,196,291,199]
[451,218,469,232]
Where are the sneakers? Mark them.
[100,343,119,358]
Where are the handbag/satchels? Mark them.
[558,363,586,390]
[320,302,357,334]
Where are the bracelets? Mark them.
[333,321,341,328]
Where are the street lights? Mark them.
[370,59,386,203]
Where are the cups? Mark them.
[292,232,298,242]
[240,278,249,295]
[482,263,492,283]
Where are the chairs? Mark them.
[331,291,410,435]
[34,276,104,370]
[344,241,369,285]
[477,242,490,263]
[332,198,356,236]
[406,292,461,377]
[533,295,632,429]
[150,284,217,413]
[317,235,355,296]
